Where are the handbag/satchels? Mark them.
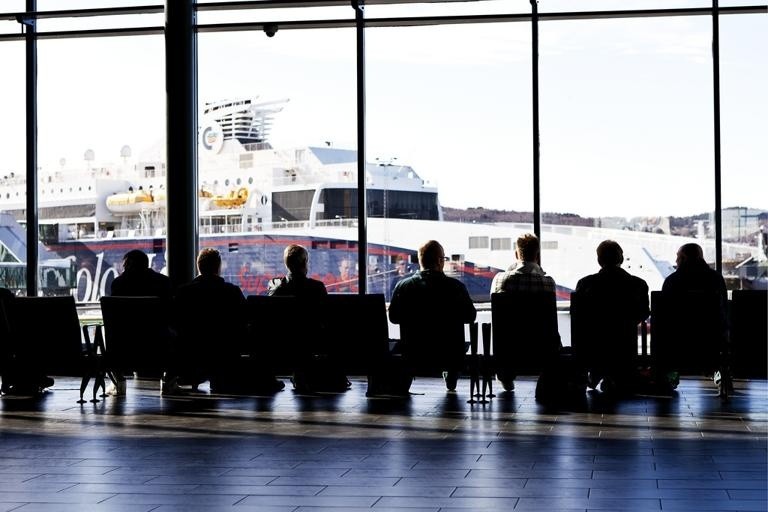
[295,353,347,394]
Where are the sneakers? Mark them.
[162,383,191,398]
[502,380,513,390]
[713,371,723,388]
[443,370,456,390]
[116,381,126,395]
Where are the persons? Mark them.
[270,243,353,391]
[387,239,477,391]
[110,248,190,396]
[489,231,558,390]
[575,240,650,391]
[660,243,737,395]
[308,250,334,292]
[388,259,409,297]
[0,286,55,387]
[180,248,287,396]
[334,255,359,293]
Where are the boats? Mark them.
[1,88,768,357]
